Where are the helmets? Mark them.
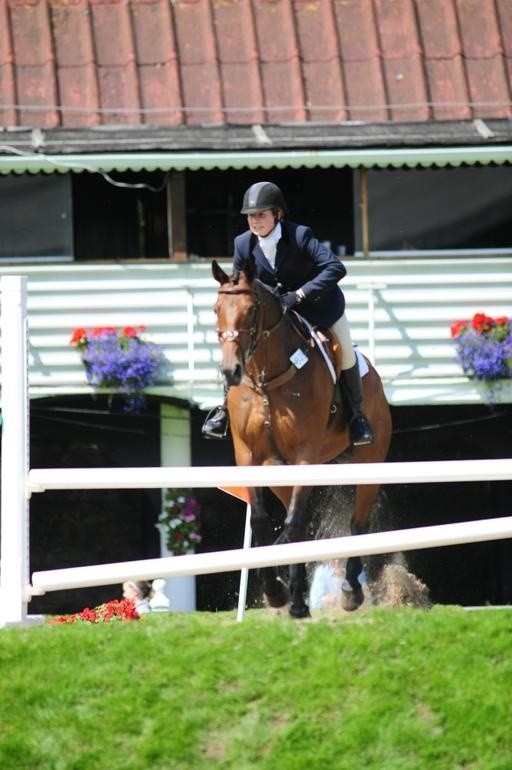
[240,181,292,216]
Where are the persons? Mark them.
[201,181,375,447]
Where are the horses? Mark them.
[208,257,395,621]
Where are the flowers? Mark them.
[451,313,510,419]
[154,489,205,557]
[67,327,164,416]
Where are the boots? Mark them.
[339,353,373,446]
[201,407,227,438]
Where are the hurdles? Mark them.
[1,275,512,623]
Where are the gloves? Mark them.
[280,287,307,310]
